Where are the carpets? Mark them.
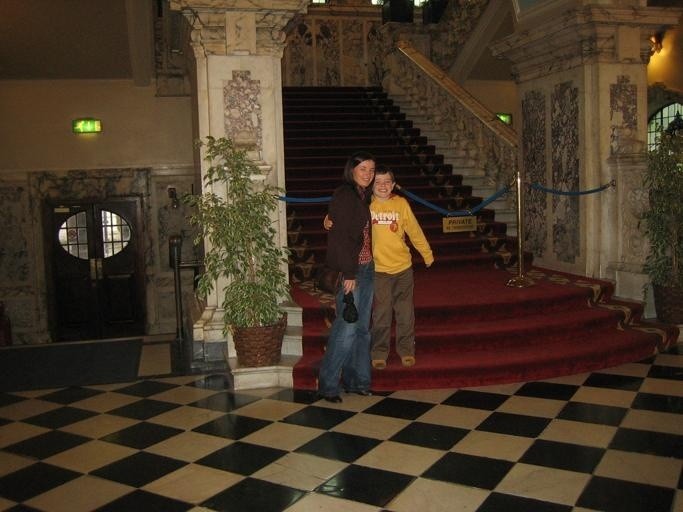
[279,86,681,391]
[1,338,143,391]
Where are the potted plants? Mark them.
[616,117,682,325]
[175,128,302,367]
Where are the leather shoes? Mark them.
[319,392,342,403]
[345,387,372,396]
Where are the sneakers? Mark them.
[402,355,415,366]
[372,360,386,370]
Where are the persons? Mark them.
[320,149,435,403]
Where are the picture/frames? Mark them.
[150,174,199,273]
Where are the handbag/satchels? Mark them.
[318,266,343,294]
